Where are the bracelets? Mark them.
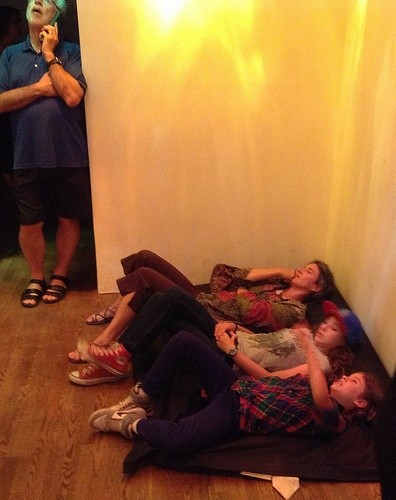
[233,322,237,331]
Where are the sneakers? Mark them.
[78,340,132,376]
[89,386,149,429]
[93,404,149,439]
[68,361,121,384]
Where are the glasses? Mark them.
[28,0,62,12]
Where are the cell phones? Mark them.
[50,13,64,33]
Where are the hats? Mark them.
[321,299,361,343]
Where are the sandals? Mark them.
[42,275,71,304]
[86,306,115,325]
[21,278,50,307]
[69,341,111,363]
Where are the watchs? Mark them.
[48,58,62,68]
[229,347,238,357]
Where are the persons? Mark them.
[0,0,88,305]
[70,286,359,380]
[67,250,334,362]
[88,330,384,455]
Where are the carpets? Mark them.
[134,277,393,487]
